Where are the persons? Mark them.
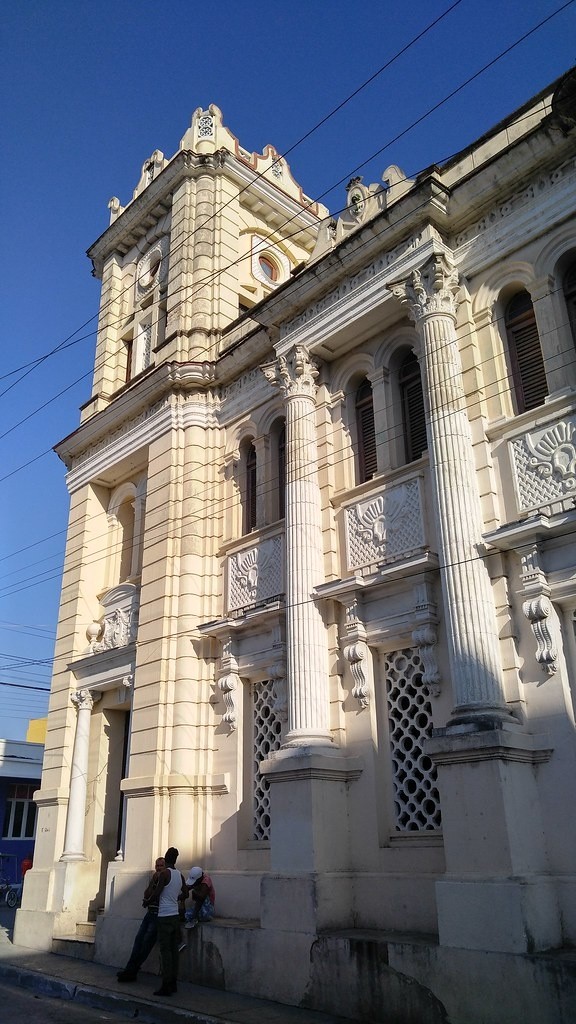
[21,852,33,896]
[179,866,216,955]
[142,847,189,996]
[116,857,165,982]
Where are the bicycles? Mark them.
[0,878,18,908]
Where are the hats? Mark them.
[185,866,202,885]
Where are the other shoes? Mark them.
[177,942,186,952]
[152,987,178,996]
[184,917,198,929]
[116,970,137,982]
